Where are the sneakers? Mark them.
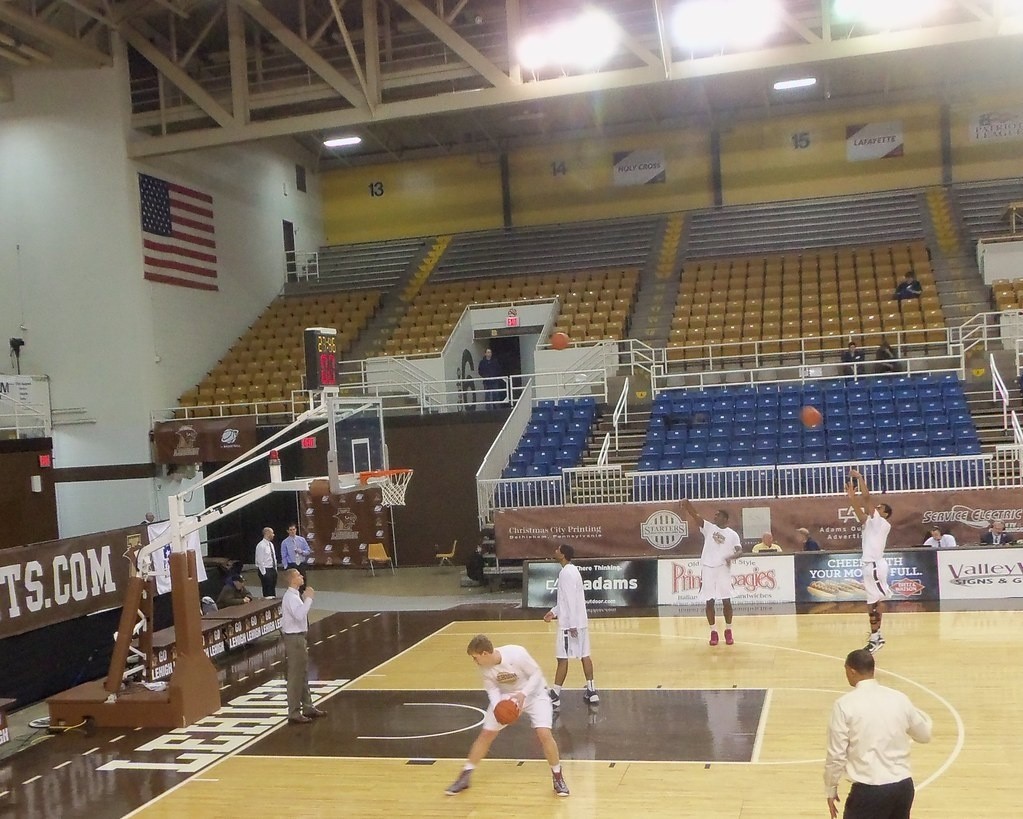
[724,629,733,644]
[863,638,885,655]
[551,766,569,796]
[583,689,599,702]
[710,631,718,645]
[548,689,560,706]
[444,769,473,796]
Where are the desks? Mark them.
[131,597,282,680]
[523,544,1023,608]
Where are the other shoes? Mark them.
[289,716,311,723]
[304,710,327,716]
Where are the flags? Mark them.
[138,172,219,293]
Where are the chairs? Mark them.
[366,543,394,577]
[174,242,1022,508]
[436,540,457,569]
[113,632,139,679]
[202,596,218,615]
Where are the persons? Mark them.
[841,342,865,376]
[141,512,156,526]
[477,346,503,409]
[444,634,570,796]
[823,650,931,819]
[979,519,1015,545]
[922,526,957,548]
[795,528,822,551]
[216,575,253,610]
[844,470,892,654]
[895,272,923,300]
[543,544,600,709]
[751,532,782,553]
[255,527,279,598]
[281,524,311,595]
[281,568,328,725]
[680,498,743,645]
[874,343,902,373]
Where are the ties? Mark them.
[995,536,998,543]
[293,539,300,565]
[300,594,309,630]
[269,543,275,569]
[938,540,941,547]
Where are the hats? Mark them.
[233,575,247,582]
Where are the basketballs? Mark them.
[494,700,519,724]
[551,332,569,351]
[800,406,823,428]
